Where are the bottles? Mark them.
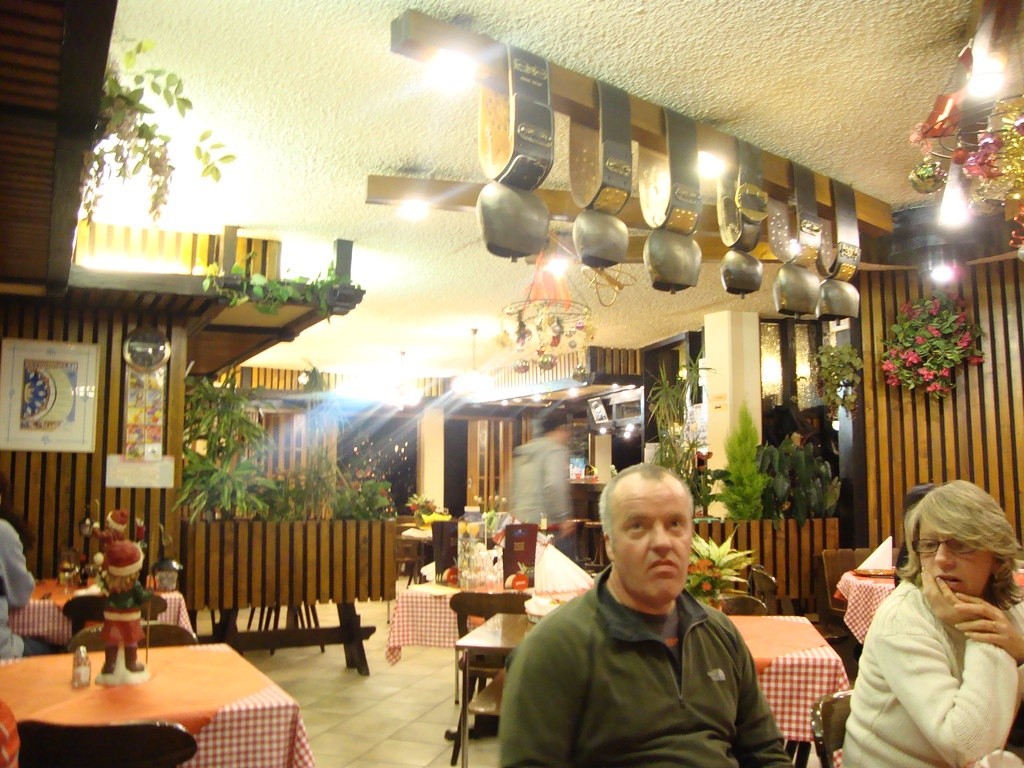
[73,645,91,688]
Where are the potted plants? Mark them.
[171,360,396,611]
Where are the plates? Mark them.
[853,569,894,577]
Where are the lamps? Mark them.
[476,44,862,326]
[452,329,482,397]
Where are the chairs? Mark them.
[63,594,195,653]
[16,720,196,768]
[394,546,903,768]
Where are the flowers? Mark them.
[683,524,757,606]
[880,287,984,401]
[405,493,438,513]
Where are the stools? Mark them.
[566,518,605,567]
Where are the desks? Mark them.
[455,613,543,768]
[724,616,851,768]
[834,565,896,665]
[386,582,594,703]
[0,576,316,768]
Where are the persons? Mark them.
[509,409,577,562]
[501,464,793,768]
[101,541,153,674]
[94,508,128,553]
[841,480,1024,768]
[0,519,68,659]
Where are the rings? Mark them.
[992,621,995,632]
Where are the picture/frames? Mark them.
[0,338,101,453]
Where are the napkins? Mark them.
[856,535,893,570]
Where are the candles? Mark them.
[541,514,547,530]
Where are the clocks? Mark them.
[123,324,171,372]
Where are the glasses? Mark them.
[913,539,978,553]
